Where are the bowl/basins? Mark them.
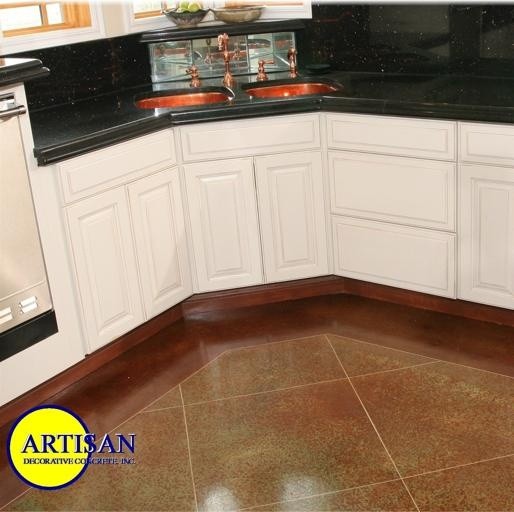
[162,6,265,26]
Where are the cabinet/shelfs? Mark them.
[50,111,514,357]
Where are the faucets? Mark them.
[216,33,236,87]
[286,47,298,78]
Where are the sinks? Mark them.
[240,78,345,97]
[132,84,235,109]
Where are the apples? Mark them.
[177,0,203,12]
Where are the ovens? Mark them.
[0,95,59,363]
